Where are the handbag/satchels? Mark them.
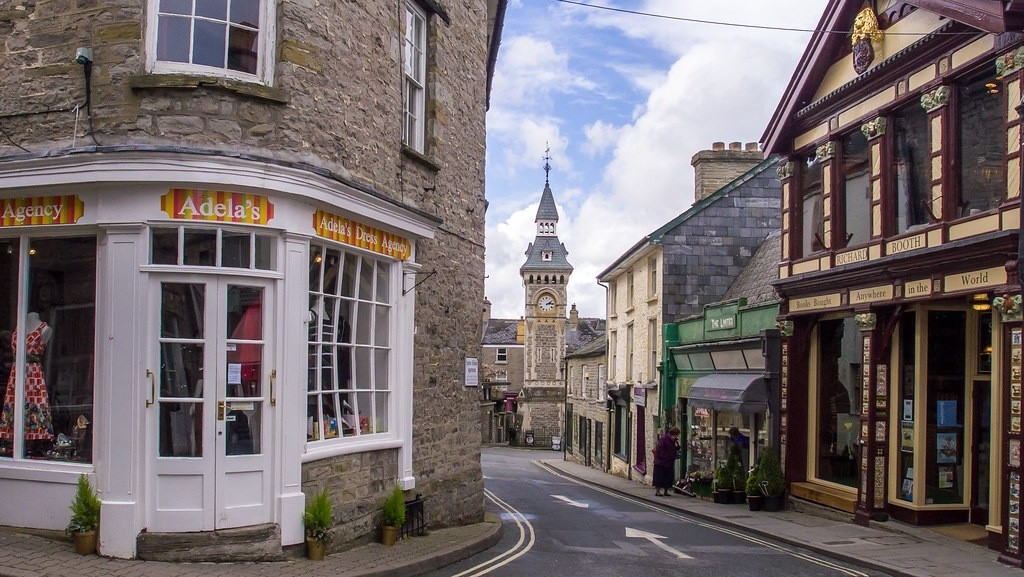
[652,447,656,454]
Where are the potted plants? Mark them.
[744,464,761,511]
[301,479,336,561]
[732,455,747,504]
[711,471,720,503]
[756,446,784,512]
[65,473,100,555]
[718,465,731,504]
[380,476,408,545]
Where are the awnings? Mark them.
[685,374,770,414]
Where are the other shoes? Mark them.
[655,492,663,496]
[664,493,671,496]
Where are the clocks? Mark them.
[538,296,556,312]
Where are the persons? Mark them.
[186,329,204,458]
[728,427,748,448]
[648,423,681,497]
[0,312,56,443]
[159,387,181,456]
[307,298,336,440]
